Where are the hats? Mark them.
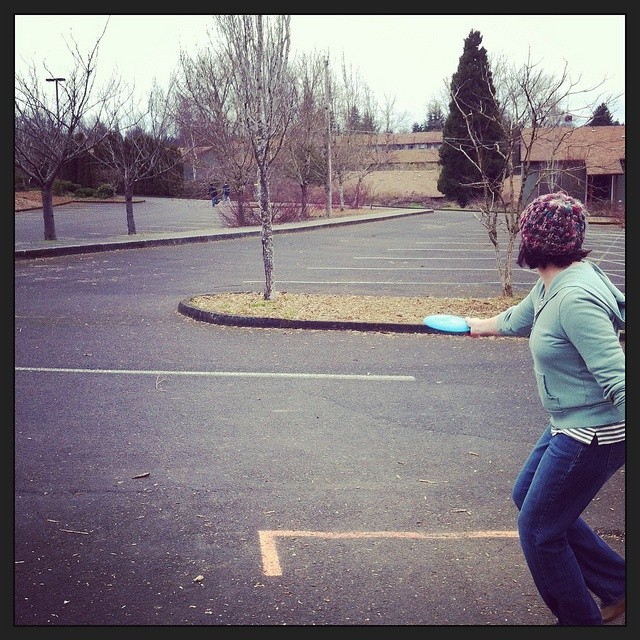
[517,189,590,255]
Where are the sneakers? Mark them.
[600,597,626,623]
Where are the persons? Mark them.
[465,195,625,626]
[222,181,231,204]
[206,183,221,207]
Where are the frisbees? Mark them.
[425,314,470,335]
